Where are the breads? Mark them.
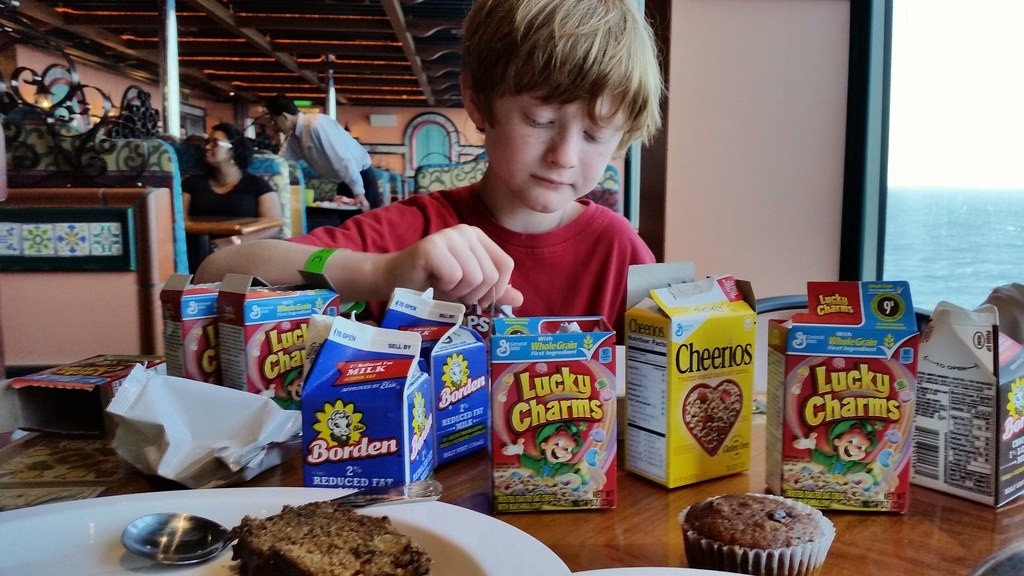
[233,500,432,576]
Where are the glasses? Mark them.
[204,140,234,148]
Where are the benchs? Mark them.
[0,150,489,373]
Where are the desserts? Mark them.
[677,492,836,576]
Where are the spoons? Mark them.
[119,476,443,564]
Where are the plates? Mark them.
[0,487,572,576]
[572,566,754,576]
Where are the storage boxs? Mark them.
[0,260,1024,513]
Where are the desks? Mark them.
[182,217,281,251]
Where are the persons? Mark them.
[181,120,282,252]
[266,95,382,220]
[191,0,662,344]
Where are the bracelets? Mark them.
[296,247,366,317]
[230,235,241,244]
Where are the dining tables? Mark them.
[0,391,1024,576]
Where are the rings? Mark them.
[214,249,217,251]
